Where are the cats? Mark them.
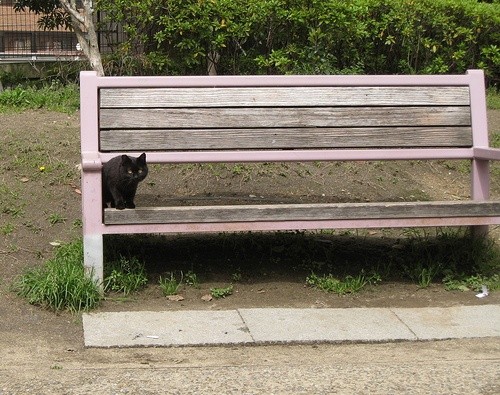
[102,152,149,210]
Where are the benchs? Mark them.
[80,69,500,300]
